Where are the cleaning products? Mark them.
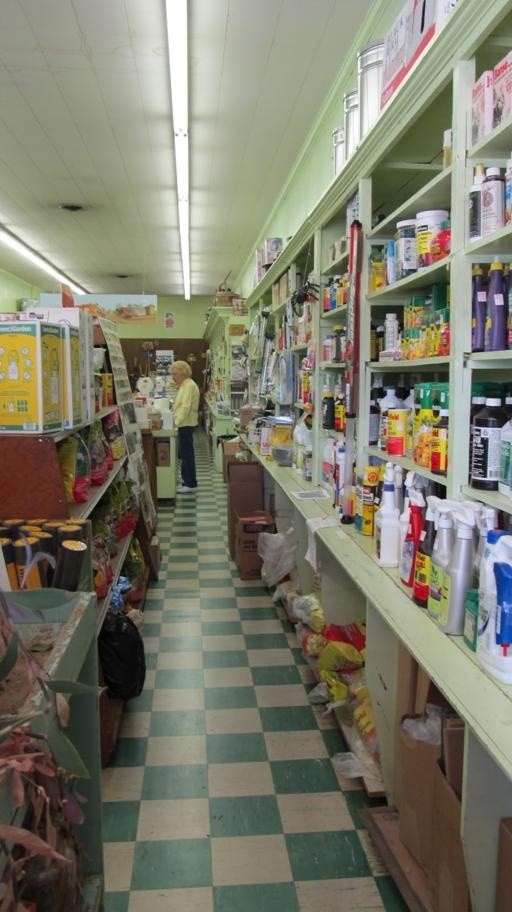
[471,256,506,350]
[377,467,474,634]
[470,395,511,490]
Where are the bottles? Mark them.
[319,161,512,535]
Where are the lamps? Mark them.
[0,223,90,294]
[164,0,191,300]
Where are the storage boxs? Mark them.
[0,307,95,432]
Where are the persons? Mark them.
[170,360,201,493]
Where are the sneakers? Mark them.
[176,483,199,494]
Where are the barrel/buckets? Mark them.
[475,530,511,683]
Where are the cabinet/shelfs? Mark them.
[460,6,512,688]
[315,533,367,703]
[361,62,455,532]
[316,176,365,507]
[263,469,315,617]
[204,210,318,487]
[366,598,399,809]
[400,645,460,910]
[0,405,149,768]
[461,721,511,911]
[0,589,104,912]
